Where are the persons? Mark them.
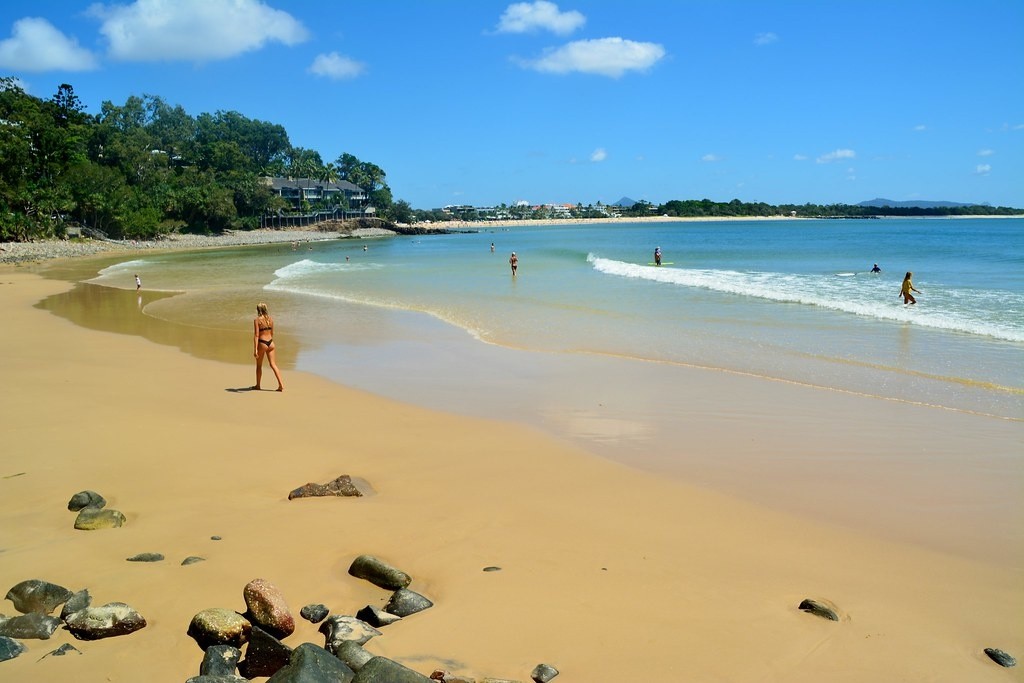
[655,247,662,266]
[871,263,882,273]
[291,239,313,251]
[508,251,519,277]
[363,245,368,251]
[250,301,285,392]
[134,274,143,296]
[491,243,495,252]
[345,256,350,263]
[899,272,922,304]
